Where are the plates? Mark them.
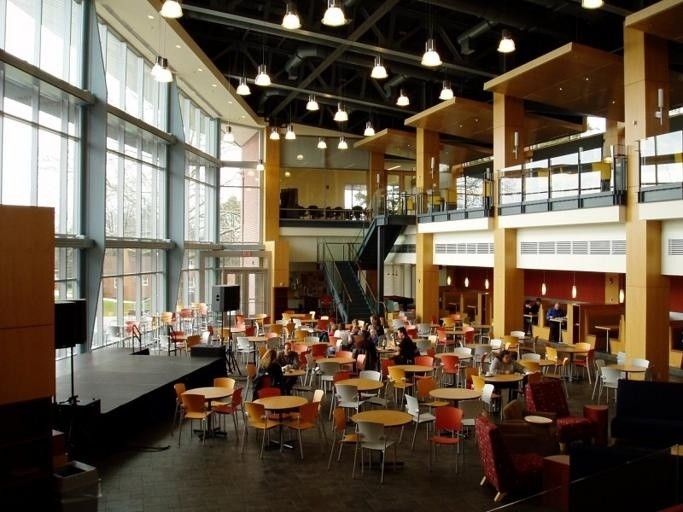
[485,375,494,377]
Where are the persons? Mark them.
[277,342,300,390]
[546,302,566,342]
[523,300,532,336]
[530,298,541,325]
[488,350,530,413]
[257,349,285,390]
[328,316,417,368]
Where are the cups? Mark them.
[487,372,493,376]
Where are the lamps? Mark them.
[445,267,625,304]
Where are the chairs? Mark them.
[298,205,365,219]
